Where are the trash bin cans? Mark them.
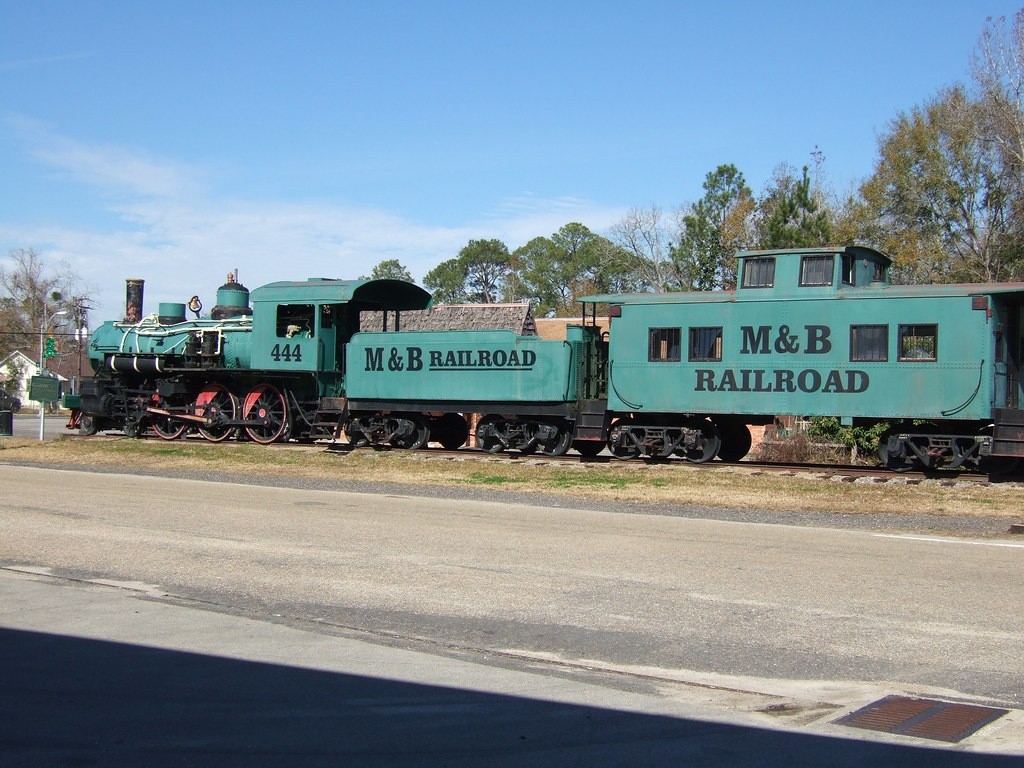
[0,411,13,436]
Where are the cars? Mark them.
[0,389,21,413]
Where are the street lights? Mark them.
[39,311,67,415]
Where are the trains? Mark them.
[62,246,1023,473]
